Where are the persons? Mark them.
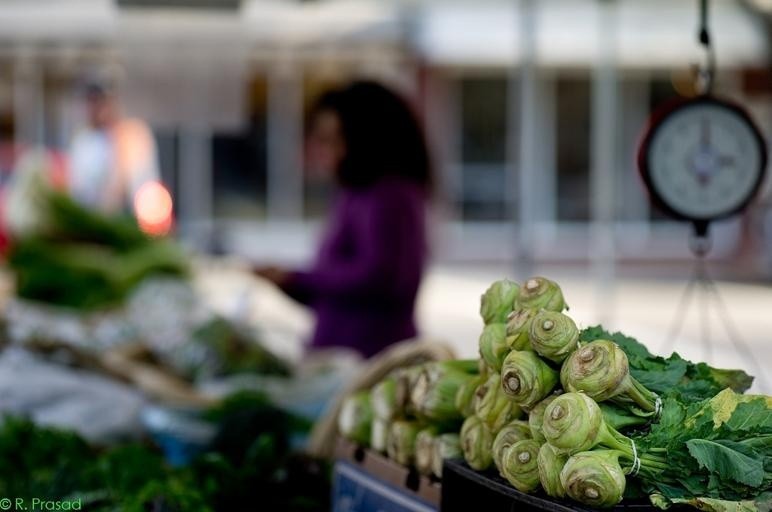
[65,68,161,221]
[253,80,432,363]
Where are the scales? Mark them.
[636,0,769,395]
